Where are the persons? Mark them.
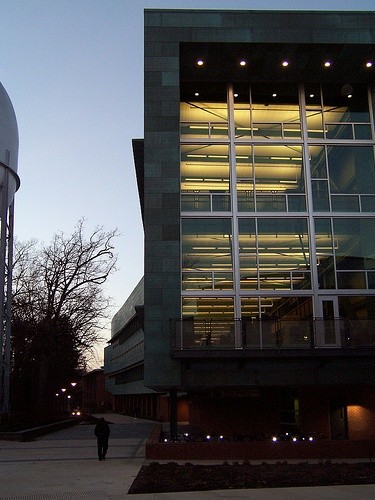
[94,417,110,461]
